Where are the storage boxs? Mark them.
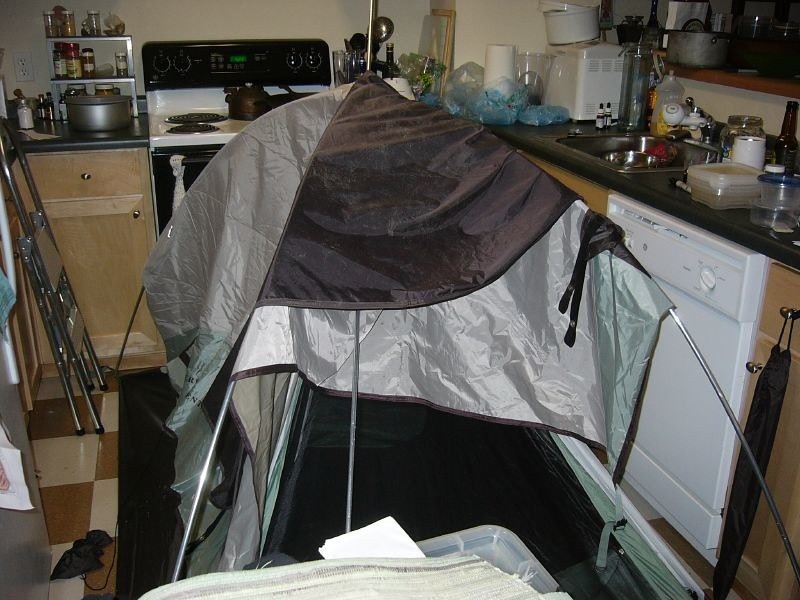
[416,523,559,595]
[686,162,765,210]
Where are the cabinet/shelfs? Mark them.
[714,257,800,600]
[0,172,42,413]
[8,146,167,379]
[45,36,139,119]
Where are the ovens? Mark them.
[151,143,227,236]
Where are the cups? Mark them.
[332,50,356,88]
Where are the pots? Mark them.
[662,30,738,70]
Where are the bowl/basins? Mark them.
[600,150,660,168]
[757,174,800,206]
[750,199,800,229]
[66,96,131,131]
[688,162,765,210]
[542,4,599,44]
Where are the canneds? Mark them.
[43,11,59,36]
[87,10,101,36]
[95,84,113,96]
[721,115,767,159]
[115,52,129,78]
[67,85,86,96]
[52,42,96,79]
[60,11,76,36]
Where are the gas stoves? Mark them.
[141,39,331,147]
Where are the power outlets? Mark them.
[13,51,34,81]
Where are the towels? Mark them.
[137,551,546,600]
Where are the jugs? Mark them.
[516,52,557,105]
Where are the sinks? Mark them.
[553,131,726,174]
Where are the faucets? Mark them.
[665,95,712,144]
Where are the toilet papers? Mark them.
[485,44,515,96]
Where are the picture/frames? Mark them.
[428,7,456,96]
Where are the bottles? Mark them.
[595,103,604,130]
[17,84,115,129]
[650,70,685,138]
[771,101,798,177]
[719,115,765,162]
[617,44,654,133]
[764,164,785,175]
[604,103,611,129]
[382,42,400,78]
[43,10,128,80]
[642,0,659,49]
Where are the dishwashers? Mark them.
[606,193,765,568]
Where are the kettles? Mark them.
[225,83,273,121]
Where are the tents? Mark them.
[116,69,800,600]
